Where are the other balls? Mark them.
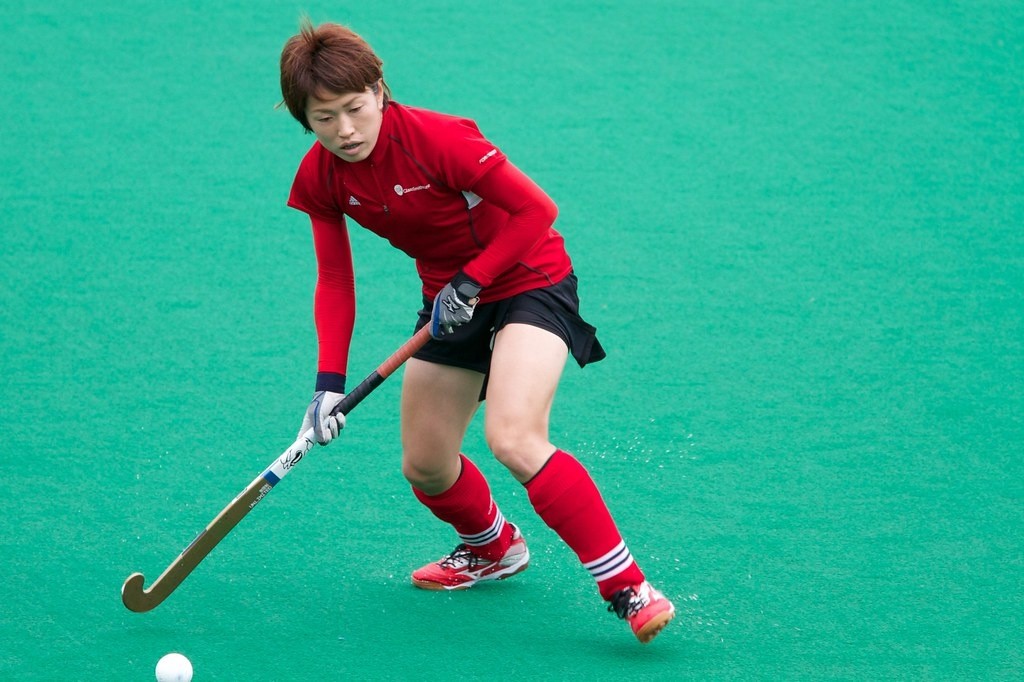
[155,653,194,682]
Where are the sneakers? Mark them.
[411,522,529,591]
[608,579,675,643]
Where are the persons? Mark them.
[282,13,675,645]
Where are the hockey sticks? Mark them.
[118,297,481,613]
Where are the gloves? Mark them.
[296,372,346,446]
[429,269,482,340]
